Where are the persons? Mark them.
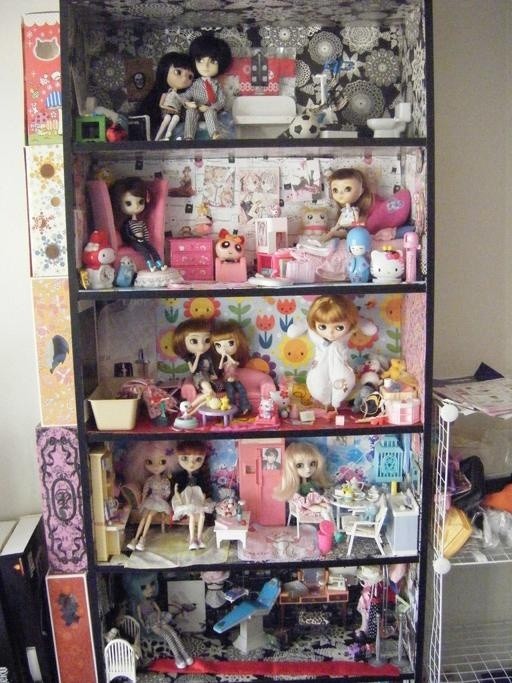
[353,565,383,642]
[122,442,174,551]
[271,443,344,543]
[145,52,194,140]
[174,444,215,551]
[115,175,171,273]
[183,35,228,141]
[211,323,254,416]
[307,171,371,245]
[119,574,194,670]
[169,318,221,409]
[305,297,359,413]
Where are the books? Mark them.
[0,513,58,683]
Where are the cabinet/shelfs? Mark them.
[424,376,512,683]
[59,1,435,682]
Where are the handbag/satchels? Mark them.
[444,456,486,559]
